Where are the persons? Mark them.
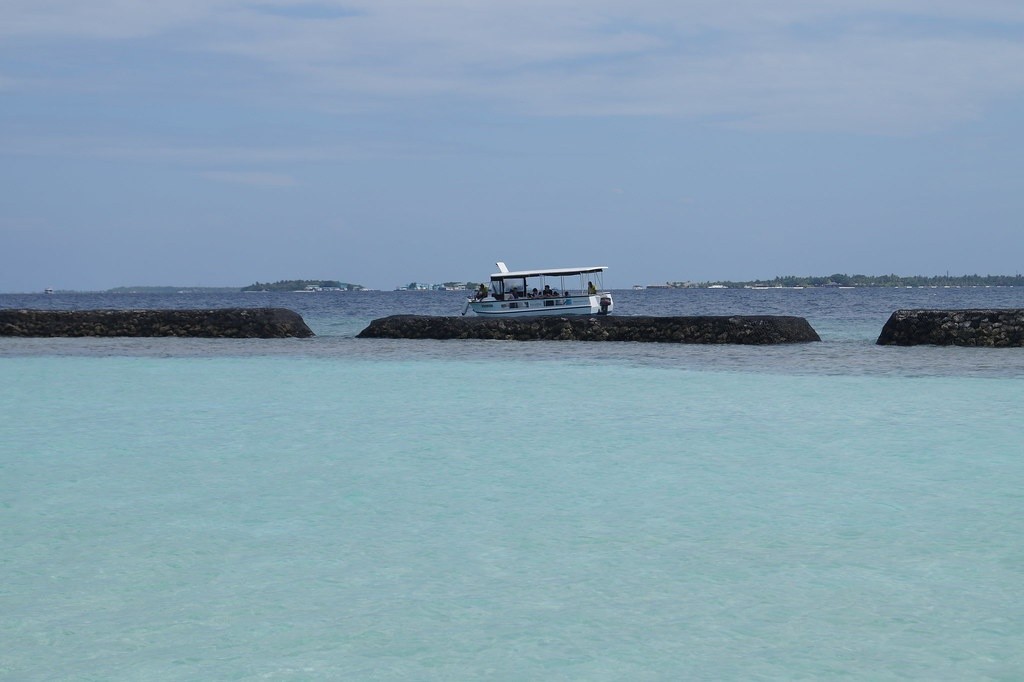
[587,281,597,296]
[509,283,573,306]
[476,284,488,302]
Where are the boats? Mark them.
[460,261,614,318]
